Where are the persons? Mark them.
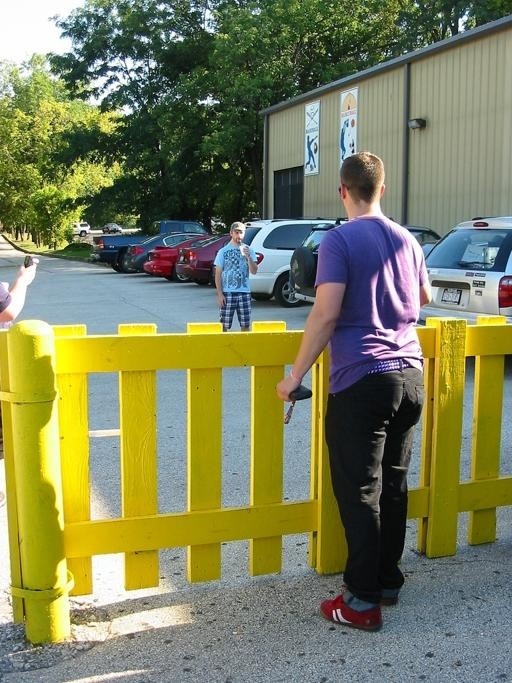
[0,256,39,509]
[305,135,318,169]
[340,121,347,160]
[275,152,433,631]
[214,221,259,332]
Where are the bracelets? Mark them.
[289,368,302,384]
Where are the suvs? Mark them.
[290,223,442,305]
[418,216,511,325]
[243,216,350,307]
[70,222,90,236]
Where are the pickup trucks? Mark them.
[93,219,211,273]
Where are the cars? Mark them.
[102,222,122,233]
[142,235,219,281]
[128,231,209,272]
[177,233,252,284]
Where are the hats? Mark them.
[230,221,246,231]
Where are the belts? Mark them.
[367,358,413,374]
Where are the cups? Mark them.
[239,245,247,256]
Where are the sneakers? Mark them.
[378,585,398,606]
[320,595,383,632]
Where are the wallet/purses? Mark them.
[289,385,312,400]
